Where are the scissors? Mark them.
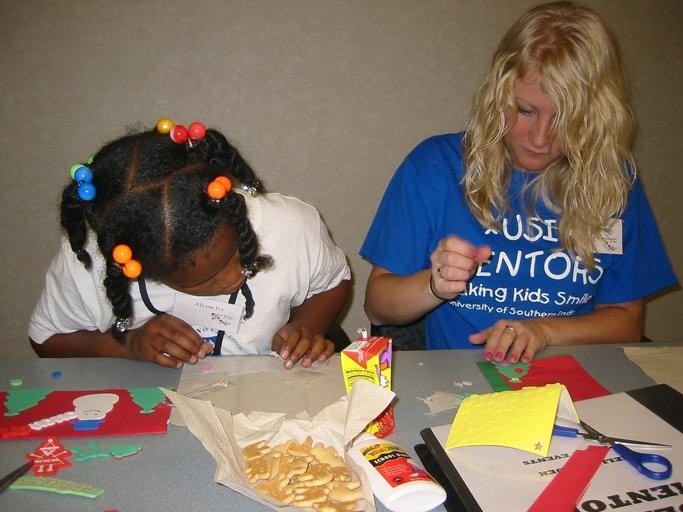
[0,459,35,493]
[547,418,674,479]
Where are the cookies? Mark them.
[240,435,361,511]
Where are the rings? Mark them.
[436,265,444,278]
[504,326,517,336]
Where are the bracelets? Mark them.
[429,273,460,302]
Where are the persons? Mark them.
[358,2,681,366]
[26,119,354,369]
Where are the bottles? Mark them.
[348,432,447,512]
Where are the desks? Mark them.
[1,341,683,512]
